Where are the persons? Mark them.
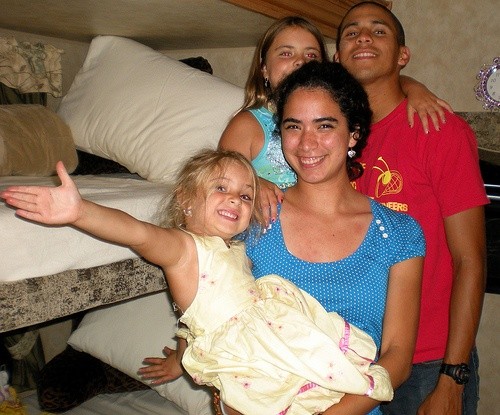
[209,59,426,415]
[0,148,394,415]
[216,14,331,228]
[333,1,491,415]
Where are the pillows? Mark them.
[71,57,213,174]
[36,349,149,413]
[56,36,245,183]
[67,290,215,415]
[0,104,79,177]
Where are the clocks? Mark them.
[473,57,500,111]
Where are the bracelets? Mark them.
[212,390,223,415]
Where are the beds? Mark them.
[1,175,173,332]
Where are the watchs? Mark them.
[440,362,471,385]
